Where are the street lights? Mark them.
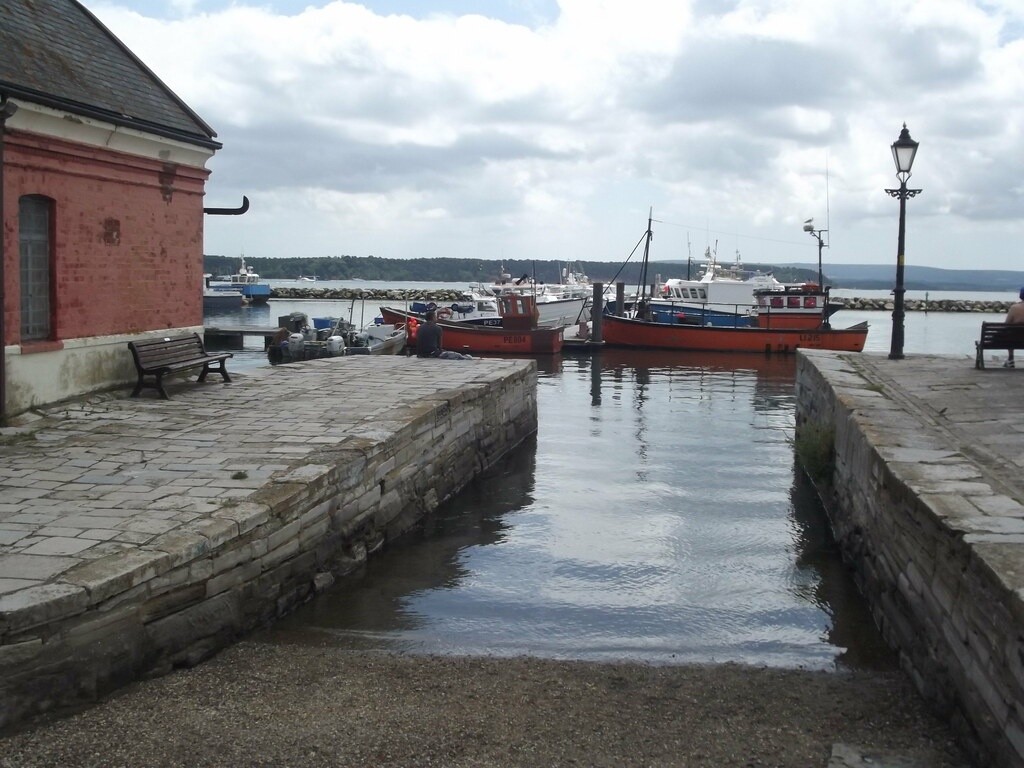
[884,122,923,359]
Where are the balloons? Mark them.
[409,317,420,337]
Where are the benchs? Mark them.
[975,321,1024,369]
[127,332,234,400]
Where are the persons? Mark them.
[417,309,472,360]
[1003,288,1024,368]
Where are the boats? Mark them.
[637,239,785,315]
[268,317,408,360]
[379,294,564,354]
[295,275,315,284]
[409,274,587,328]
[590,205,869,353]
[203,257,270,305]
